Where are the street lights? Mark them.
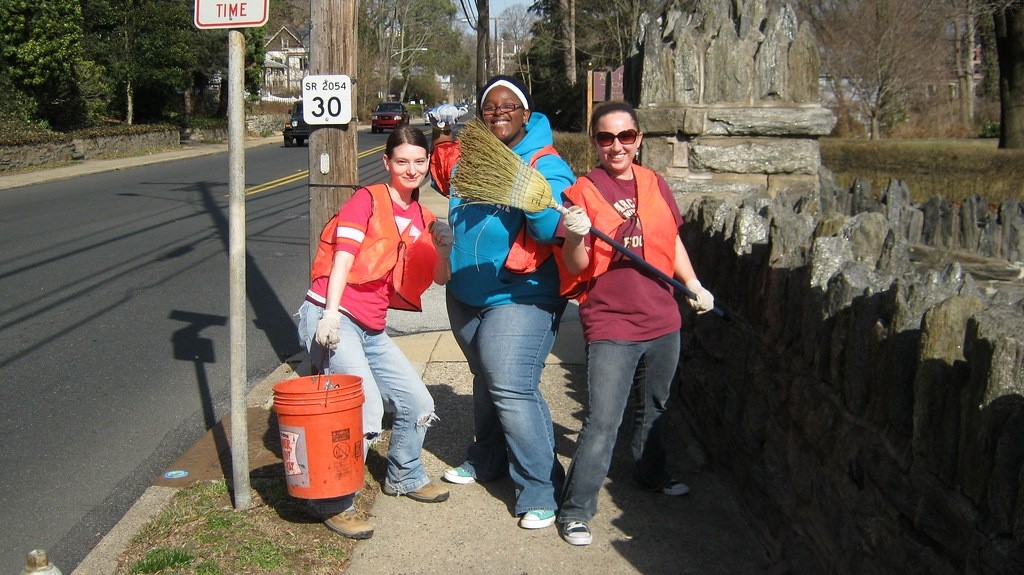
[387,48,427,102]
[461,18,499,75]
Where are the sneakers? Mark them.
[382,477,449,503]
[322,505,374,540]
[444,466,480,484]
[560,519,593,545]
[661,478,690,496]
[520,510,559,529]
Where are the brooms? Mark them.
[447,114,737,325]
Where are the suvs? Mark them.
[283,100,309,148]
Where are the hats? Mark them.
[478,74,533,126]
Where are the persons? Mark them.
[292,124,454,540]
[445,73,578,530]
[555,102,714,547]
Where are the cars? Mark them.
[424,106,438,125]
[371,102,409,133]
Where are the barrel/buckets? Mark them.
[271,340,365,499]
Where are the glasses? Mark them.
[483,103,521,115]
[593,128,638,147]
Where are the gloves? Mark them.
[314,310,342,350]
[428,220,454,259]
[429,104,469,131]
[563,205,592,244]
[684,277,715,316]
